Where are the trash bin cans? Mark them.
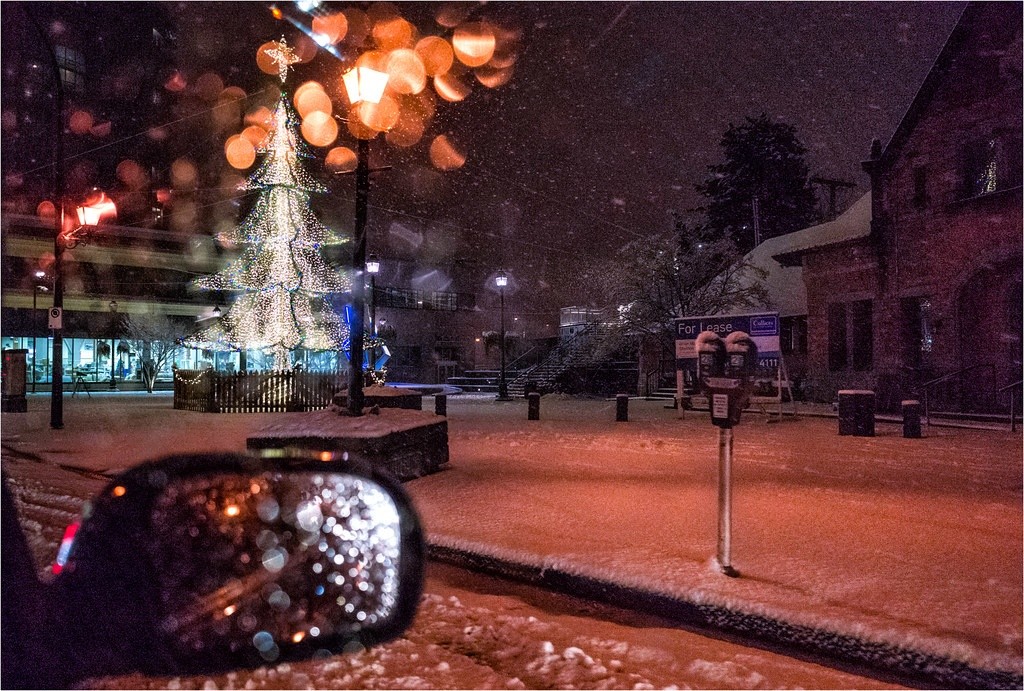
[839,389,875,437]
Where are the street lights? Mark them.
[364,252,383,385]
[49,198,100,431]
[494,268,513,401]
[31,270,46,393]
[108,298,118,391]
[339,47,390,418]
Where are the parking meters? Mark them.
[695,331,759,578]
